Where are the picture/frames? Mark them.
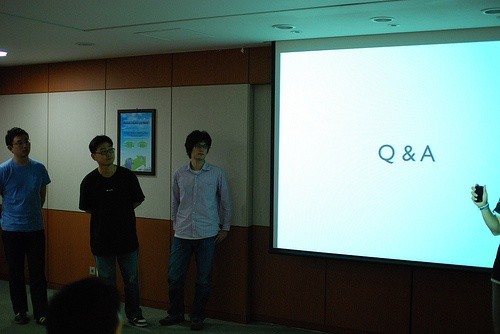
[117,109,155,175]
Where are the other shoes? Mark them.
[36,316,46,325]
[14,313,28,321]
[190,316,204,330]
[127,316,149,327]
[158,314,185,326]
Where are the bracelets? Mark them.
[480,203,489,212]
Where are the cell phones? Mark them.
[474,186,483,203]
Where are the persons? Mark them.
[0,126,51,326]
[159,130,231,331]
[78,135,149,327]
[45,275,125,334]
[471,185,500,334]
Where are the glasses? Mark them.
[195,144,208,149]
[94,148,115,155]
